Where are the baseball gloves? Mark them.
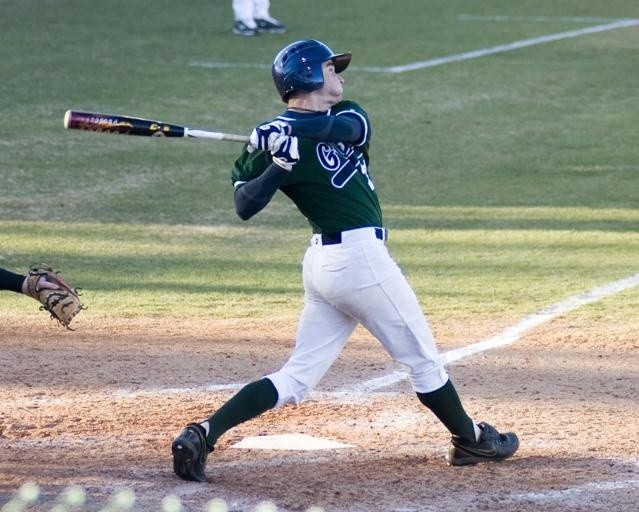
[27,262,88,332]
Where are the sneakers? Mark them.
[444,421,520,468]
[170,421,215,484]
[231,15,287,37]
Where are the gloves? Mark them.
[245,118,302,172]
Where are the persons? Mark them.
[170,35,521,480]
[1,254,83,328]
[227,0,288,36]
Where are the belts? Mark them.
[319,227,385,247]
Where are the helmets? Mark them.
[270,38,354,104]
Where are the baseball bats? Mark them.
[63,110,250,142]
